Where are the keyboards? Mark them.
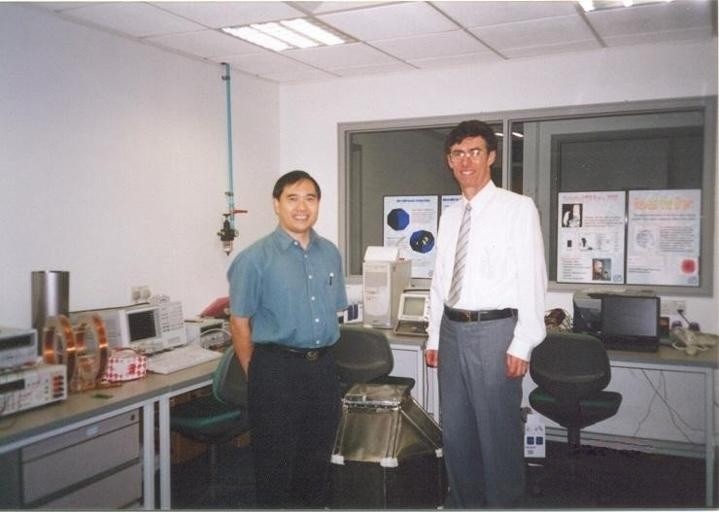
[145,342,223,376]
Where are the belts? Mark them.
[257,342,322,362]
[444,304,515,323]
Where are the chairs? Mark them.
[336,325,416,404]
[527,335,623,502]
[154,345,253,510]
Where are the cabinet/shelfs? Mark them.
[2,405,143,512]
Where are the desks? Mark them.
[102,343,230,510]
[370,326,429,412]
[433,329,718,505]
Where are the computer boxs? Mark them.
[362,259,411,330]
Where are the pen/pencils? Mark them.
[330,273,334,285]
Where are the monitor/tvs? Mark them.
[397,293,428,322]
[119,304,161,352]
[572,294,660,353]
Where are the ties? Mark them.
[447,203,471,308]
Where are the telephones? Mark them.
[673,328,716,347]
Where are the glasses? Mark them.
[450,148,488,161]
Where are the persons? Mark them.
[421,120,549,508]
[226,169,346,510]
